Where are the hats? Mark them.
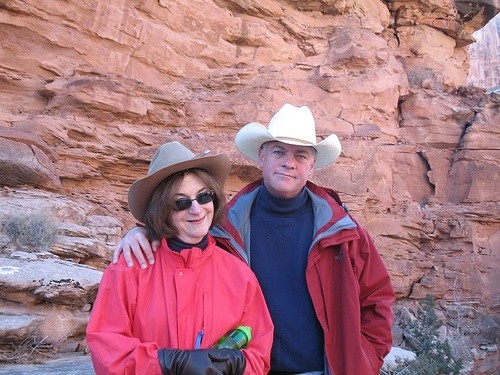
[128,141,231,223]
[235,104,341,169]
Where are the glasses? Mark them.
[175,190,215,211]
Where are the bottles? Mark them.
[211,325,253,352]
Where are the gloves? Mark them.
[213,350,246,375]
[158,348,229,375]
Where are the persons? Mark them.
[113,103,395,375]
[85,141,274,375]
